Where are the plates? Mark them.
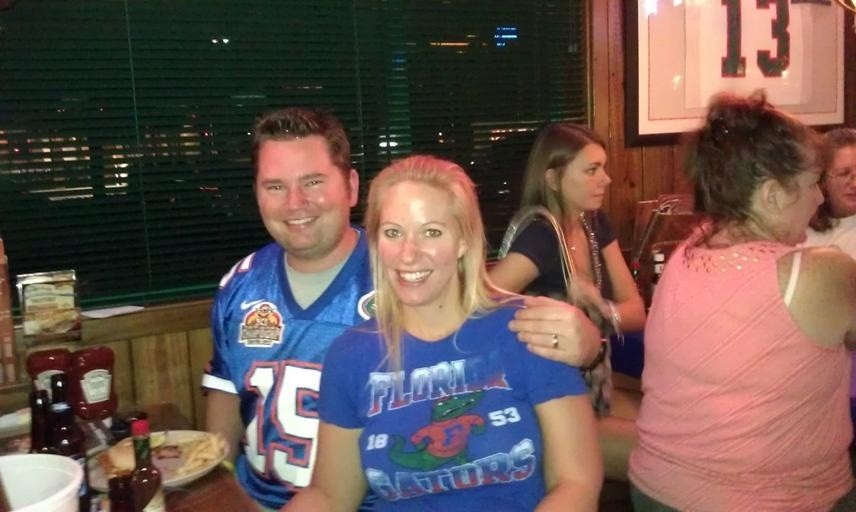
[89,426,232,490]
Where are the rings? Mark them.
[552,334,559,349]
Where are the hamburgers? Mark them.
[99,436,136,481]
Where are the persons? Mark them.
[285,155,604,510]
[488,123,646,408]
[200,107,601,512]
[811,128,856,261]
[628,91,856,510]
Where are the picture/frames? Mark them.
[622,0,856,148]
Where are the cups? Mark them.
[0,453,85,512]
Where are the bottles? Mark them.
[28,345,165,512]
[653,253,666,282]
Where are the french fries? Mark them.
[177,429,228,475]
